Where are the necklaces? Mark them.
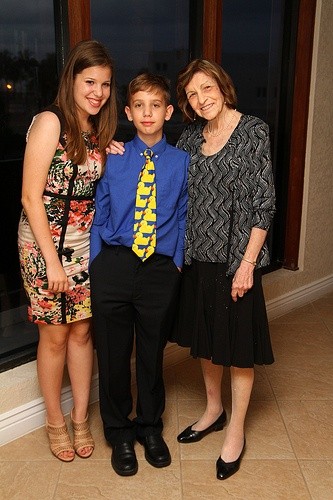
[208,108,237,137]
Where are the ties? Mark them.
[131,149,157,262]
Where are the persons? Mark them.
[90,70,190,476]
[18,40,118,463]
[107,56,277,481]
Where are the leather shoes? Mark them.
[137,434,171,468]
[111,441,139,477]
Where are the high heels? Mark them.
[216,435,246,480]
[177,408,226,443]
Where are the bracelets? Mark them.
[241,257,258,266]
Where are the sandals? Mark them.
[71,407,95,458]
[46,417,75,462]
